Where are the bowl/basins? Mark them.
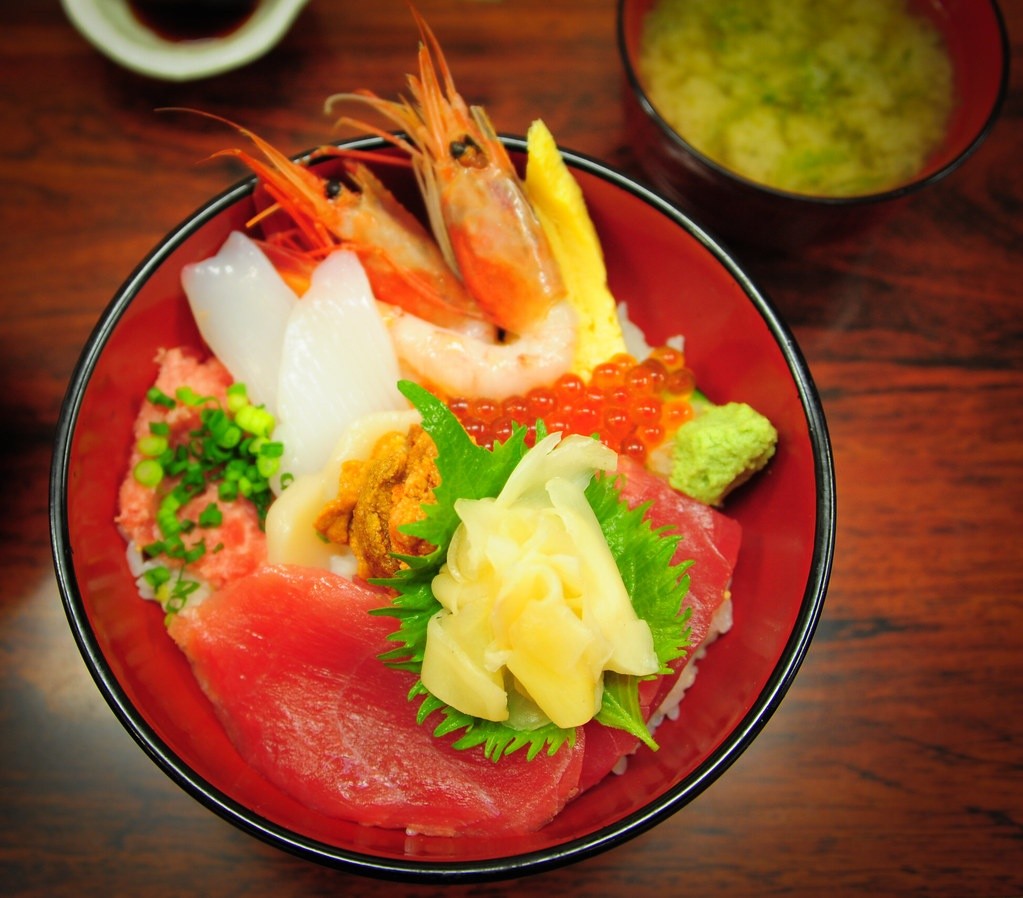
[615,0,1011,208]
[51,129,835,885]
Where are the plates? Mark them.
[58,0,306,83]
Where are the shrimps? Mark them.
[179,7,574,410]
